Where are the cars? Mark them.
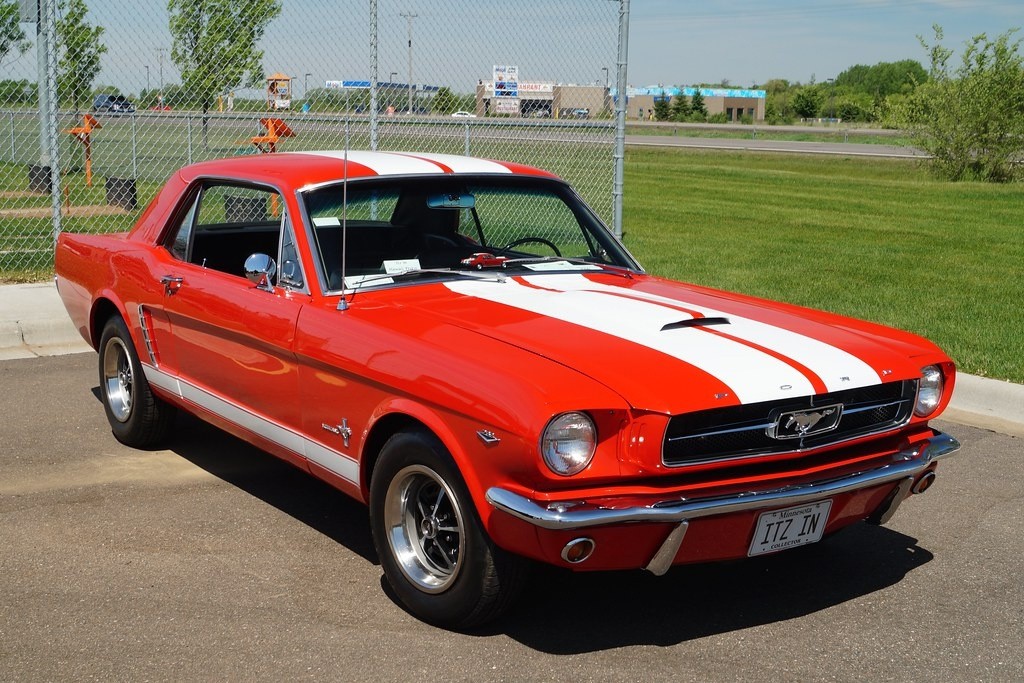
[394,106,427,115]
[147,105,170,110]
[558,108,589,120]
[55,150,961,630]
[92,94,135,118]
[452,111,477,118]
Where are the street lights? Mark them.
[390,73,397,83]
[144,66,150,110]
[602,68,608,89]
[291,76,297,117]
[827,78,833,118]
[305,74,310,102]
